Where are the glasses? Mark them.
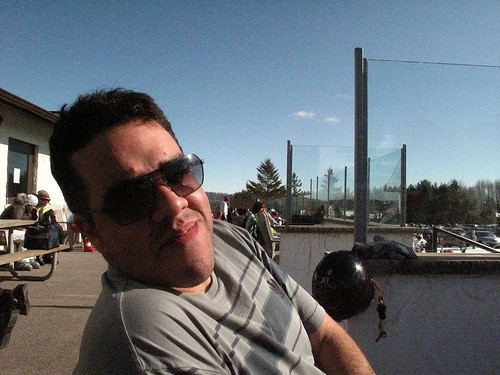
[82,153,205,226]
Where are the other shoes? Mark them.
[44,254,60,265]
[17,262,33,271]
[36,257,46,265]
[30,261,41,269]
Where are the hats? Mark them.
[38,190,51,199]
[27,194,38,205]
[269,208,276,215]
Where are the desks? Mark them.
[1,219,35,280]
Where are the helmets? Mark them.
[312,250,376,321]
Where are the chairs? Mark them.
[258,212,280,264]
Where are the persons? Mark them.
[220,194,231,221]
[0,283,30,349]
[231,201,281,241]
[49,89,376,375]
[0,194,40,270]
[25,190,61,266]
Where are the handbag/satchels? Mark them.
[25,223,60,250]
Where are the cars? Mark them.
[447,223,500,249]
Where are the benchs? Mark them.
[0,244,71,281]
